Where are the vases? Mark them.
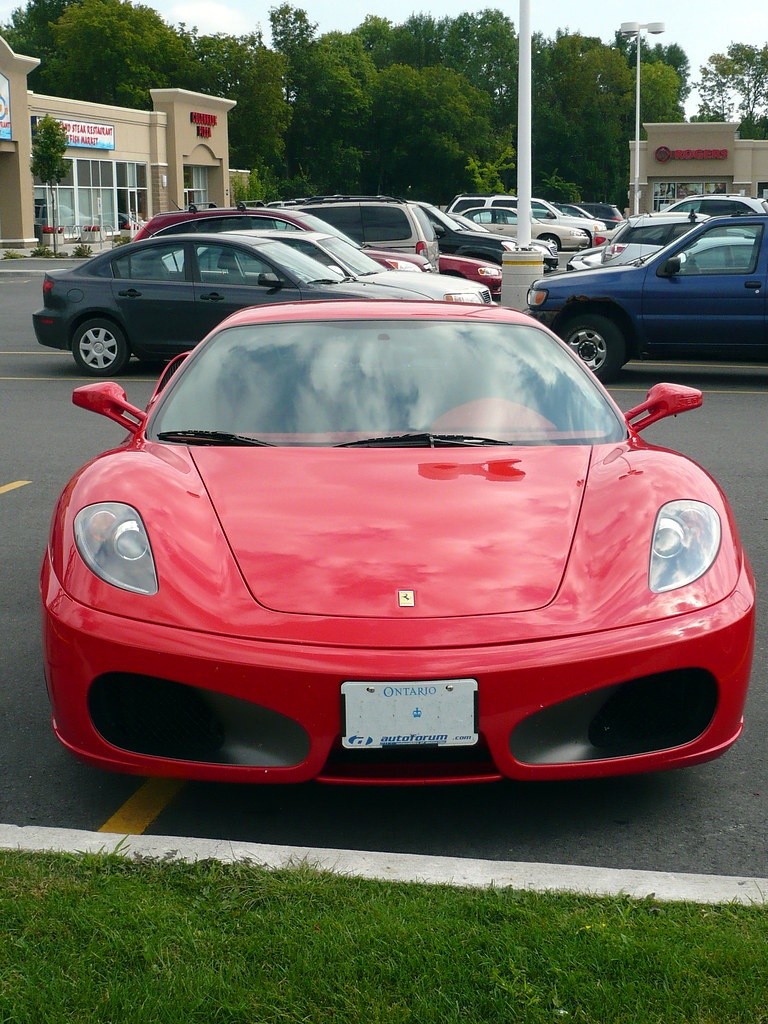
[81,231,105,243]
[42,234,64,246]
[120,230,140,243]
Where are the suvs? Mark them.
[124,203,435,280]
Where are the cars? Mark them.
[260,195,626,298]
[154,229,502,312]
[566,194,767,278]
[31,231,458,378]
[523,210,767,384]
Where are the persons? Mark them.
[712,184,726,193]
[654,183,668,196]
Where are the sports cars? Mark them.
[38,299,758,799]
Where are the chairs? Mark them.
[498,214,504,224]
[217,248,247,284]
[503,214,508,224]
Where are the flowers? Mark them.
[83,225,101,232]
[124,224,139,230]
[41,226,65,234]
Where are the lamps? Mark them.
[162,175,167,188]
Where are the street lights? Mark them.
[621,21,666,216]
